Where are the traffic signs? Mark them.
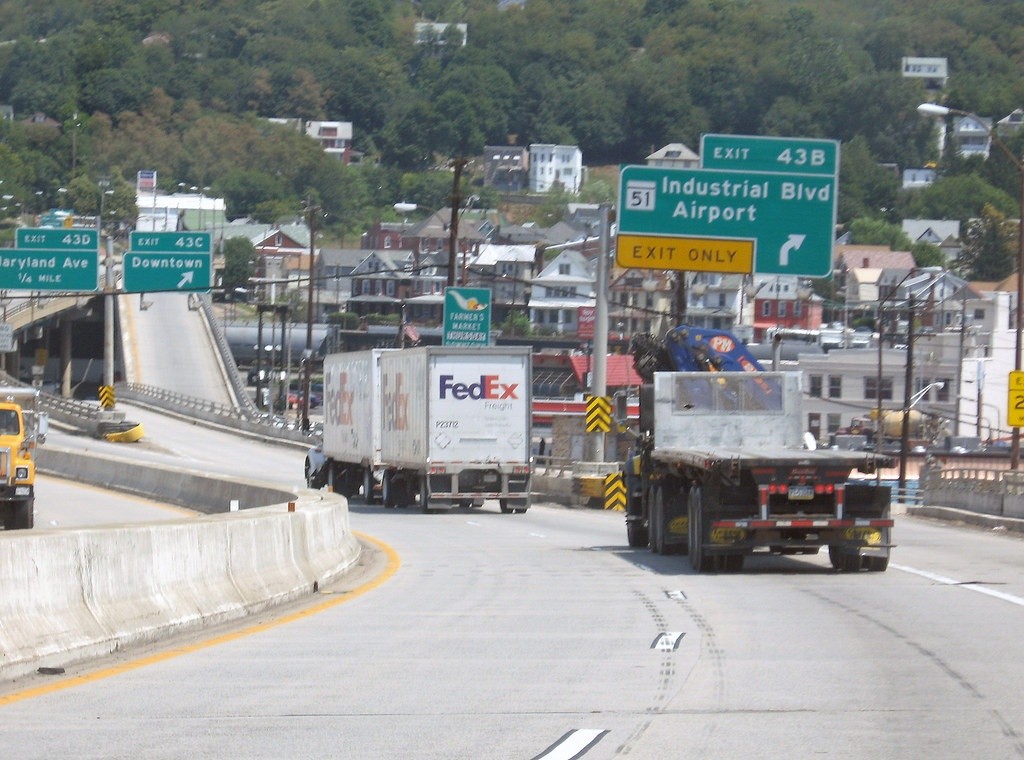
[0,228,102,294]
[122,230,211,295]
[616,132,842,281]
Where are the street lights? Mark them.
[918,101,1021,470]
[898,378,948,504]
[391,194,483,287]
[100,190,116,218]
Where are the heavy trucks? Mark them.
[612,369,898,576]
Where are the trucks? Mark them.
[303,344,535,516]
[0,383,50,530]
